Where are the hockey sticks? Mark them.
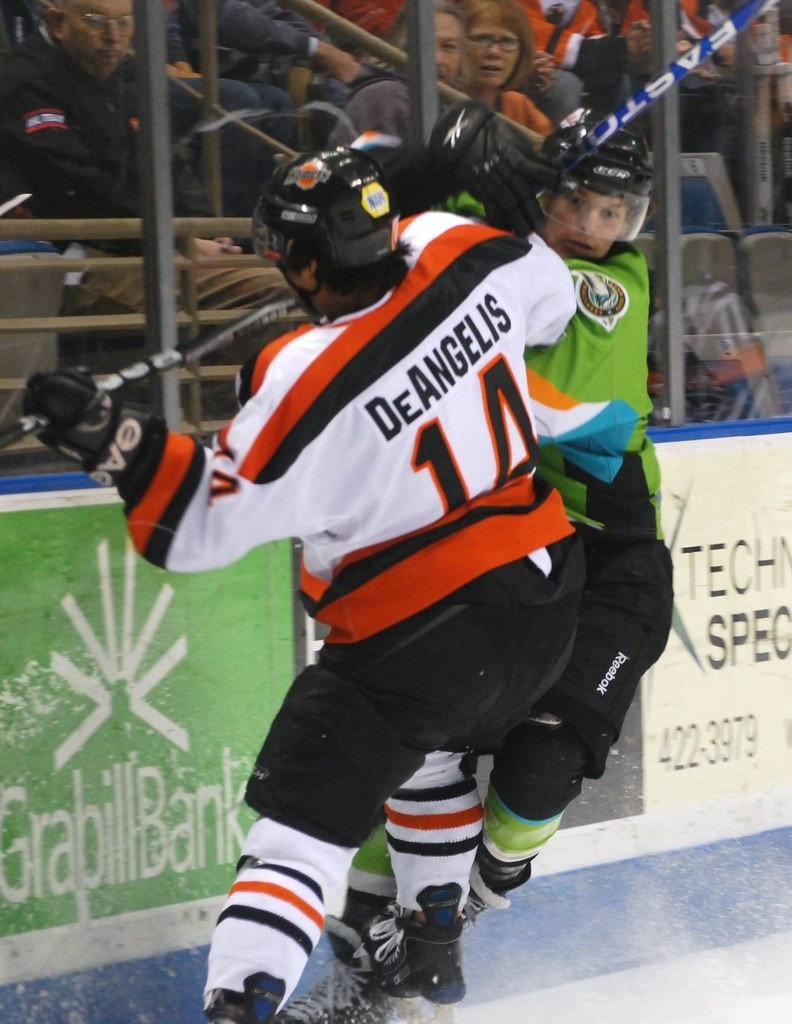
[552,1,786,179]
[0,295,306,452]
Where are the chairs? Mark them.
[1,152,792,477]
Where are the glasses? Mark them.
[82,13,133,30]
[468,34,527,52]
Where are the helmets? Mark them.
[257,146,394,267]
[542,108,651,198]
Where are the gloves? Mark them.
[21,370,163,488]
[428,101,560,236]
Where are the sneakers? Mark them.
[462,861,532,925]
[276,915,392,1023]
[363,915,466,1004]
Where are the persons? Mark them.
[19,109,674,1024]
[0,0,792,446]
